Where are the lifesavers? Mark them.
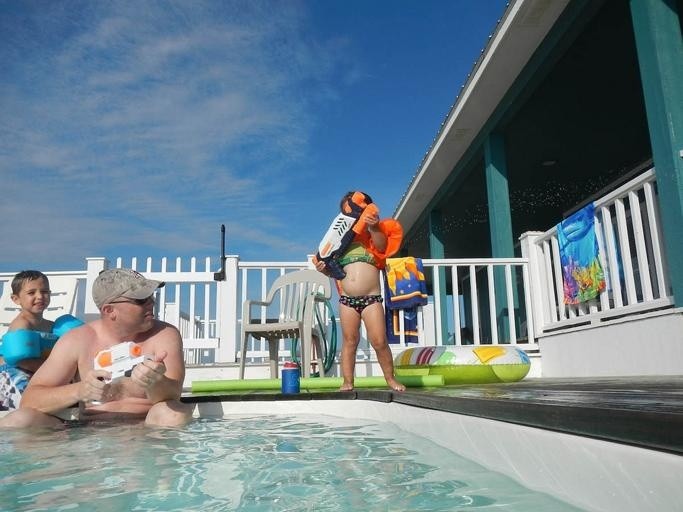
[393,345,532,385]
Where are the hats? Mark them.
[92,269,165,310]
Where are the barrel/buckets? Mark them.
[281,362,301,394]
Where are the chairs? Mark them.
[238,269,332,379]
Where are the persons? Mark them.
[0,270,55,411]
[0,267,191,427]
[15,437,174,511]
[315,191,407,392]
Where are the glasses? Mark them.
[110,298,149,305]
